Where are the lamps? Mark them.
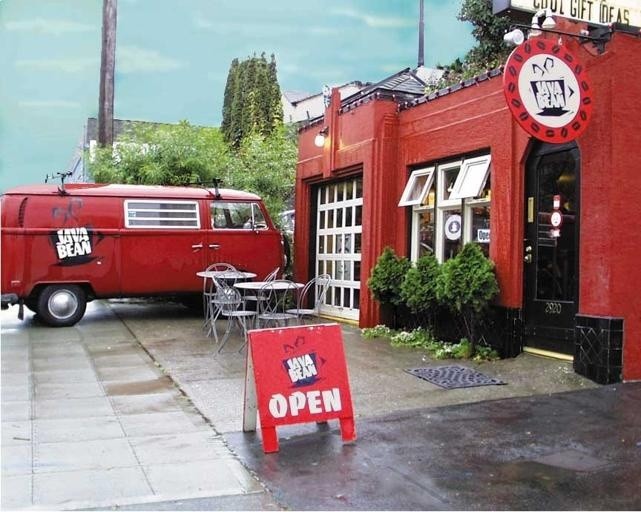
[503,22,609,54]
[314,126,329,147]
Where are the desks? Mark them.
[195,271,257,328]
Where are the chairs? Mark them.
[201,262,332,355]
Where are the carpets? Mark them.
[401,364,509,391]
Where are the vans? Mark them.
[0,170,291,327]
